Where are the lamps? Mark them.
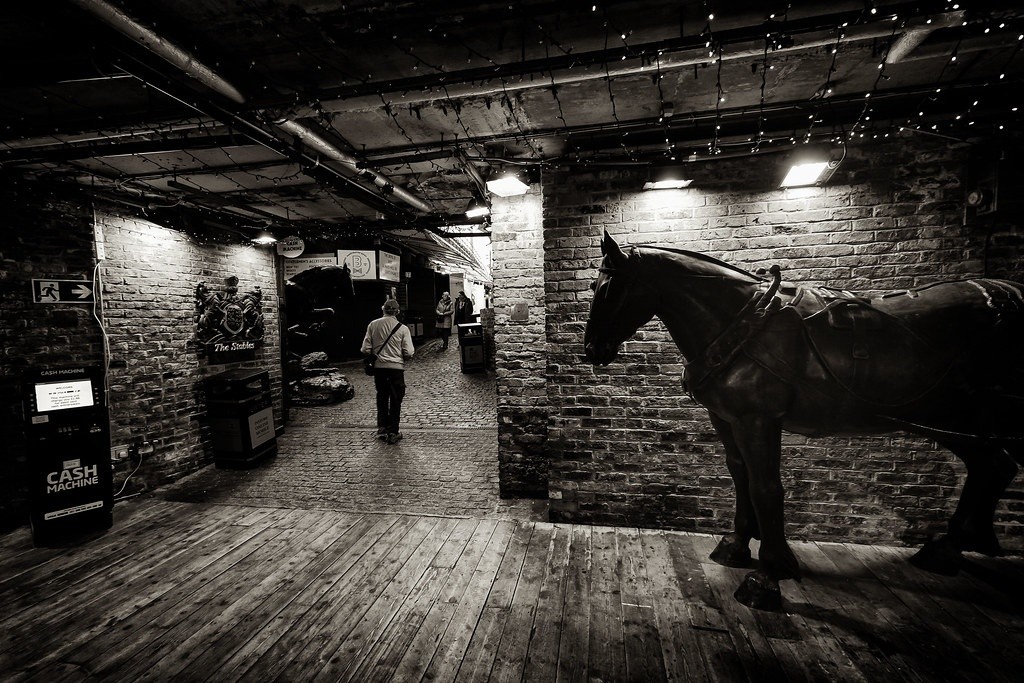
[642,165,693,189]
[464,197,491,219]
[486,173,531,198]
[775,149,833,189]
[251,230,277,245]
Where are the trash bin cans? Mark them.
[202,368,280,470]
[405,316,424,345]
[458,324,485,372]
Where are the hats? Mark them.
[382,300,398,313]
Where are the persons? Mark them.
[435,292,454,351]
[360,299,414,445]
[454,291,474,326]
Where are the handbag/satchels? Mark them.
[364,355,376,375]
[437,315,444,324]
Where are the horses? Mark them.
[582,221,1024,614]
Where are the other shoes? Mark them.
[387,433,402,444]
[378,426,387,437]
